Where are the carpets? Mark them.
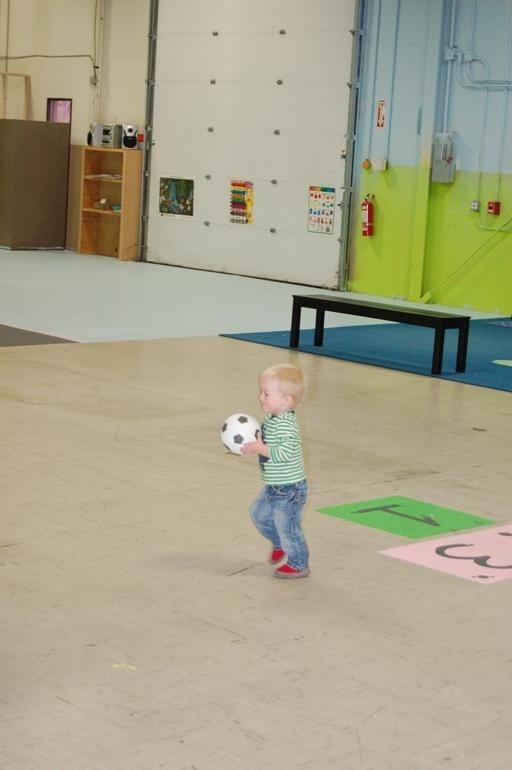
[218,307,511,393]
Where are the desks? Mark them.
[288,292,472,378]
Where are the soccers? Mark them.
[220,414,263,458]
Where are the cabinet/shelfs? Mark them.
[0,116,74,252]
[76,143,145,262]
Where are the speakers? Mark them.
[102,124,122,148]
[87,122,102,147]
[122,123,137,149]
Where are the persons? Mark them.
[240,362,310,580]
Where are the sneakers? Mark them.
[273,564,308,579]
[271,547,286,563]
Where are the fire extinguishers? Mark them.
[360,193,376,236]
